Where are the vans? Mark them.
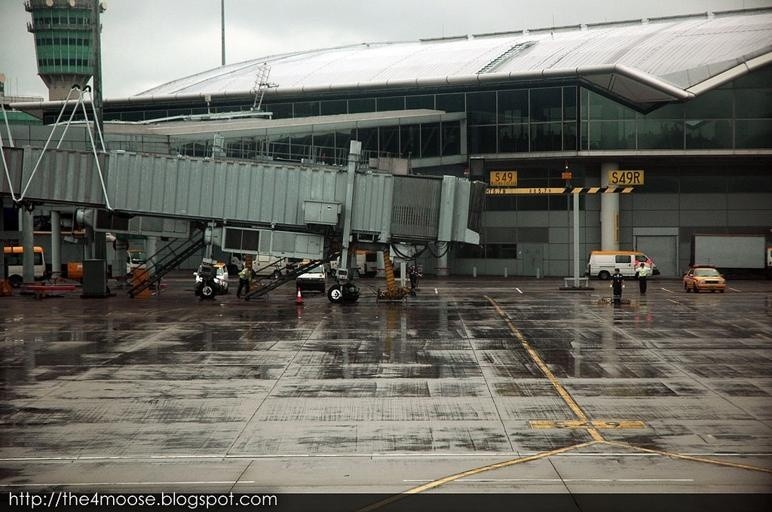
[190,260,230,292]
[245,254,289,280]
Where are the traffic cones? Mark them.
[294,302,304,320]
[294,287,305,304]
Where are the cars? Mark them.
[679,266,729,293]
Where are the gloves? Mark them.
[609,285,612,288]
[622,285,626,288]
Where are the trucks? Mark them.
[125,247,146,273]
[328,251,379,278]
[583,250,662,282]
[688,232,772,280]
[2,245,49,290]
[294,261,325,294]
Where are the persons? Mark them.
[612,268,623,297]
[635,262,649,295]
[321,150,328,163]
[237,265,252,300]
